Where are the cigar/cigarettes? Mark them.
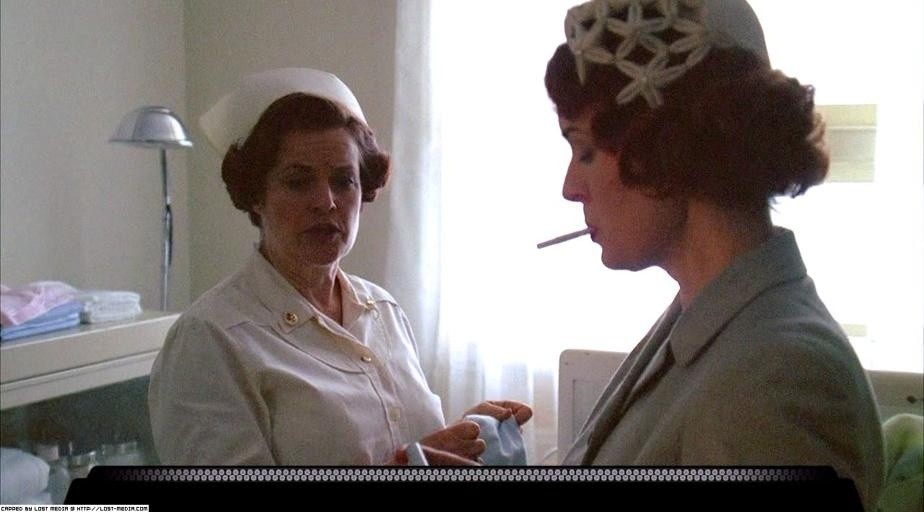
[537,227,591,249]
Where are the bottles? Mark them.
[36,434,144,504]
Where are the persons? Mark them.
[394,0,885,511]
[146,67,532,468]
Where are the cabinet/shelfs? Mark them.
[1,311,183,505]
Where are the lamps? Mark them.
[106,106,196,309]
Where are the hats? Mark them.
[565,1,770,108]
[198,67,369,159]
